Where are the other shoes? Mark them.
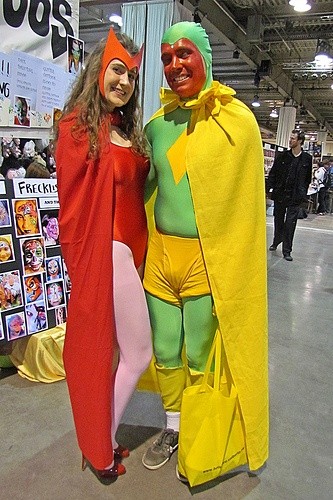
[319,211,326,215]
[283,253,293,261]
[269,241,281,251]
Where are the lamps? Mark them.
[329,72,333,80]
[330,135,332,137]
[327,133,330,136]
[311,72,318,80]
[233,47,241,59]
[286,19,295,32]
[320,72,328,81]
[322,125,325,129]
[302,72,308,81]
[251,95,261,108]
[269,107,279,118]
[331,101,333,105]
[295,121,301,129]
[192,7,205,23]
[320,102,323,105]
[324,101,326,105]
[294,72,299,79]
[327,100,330,106]
[288,0,312,13]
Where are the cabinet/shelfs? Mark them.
[263,140,288,199]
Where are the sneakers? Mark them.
[142,428,180,470]
[175,464,189,482]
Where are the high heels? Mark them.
[81,453,126,477]
[114,444,130,457]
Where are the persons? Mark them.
[138,21,269,488]
[266,129,312,260]
[69,40,82,74]
[54,30,153,478]
[14,96,30,126]
[314,159,333,214]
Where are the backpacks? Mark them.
[320,168,329,183]
